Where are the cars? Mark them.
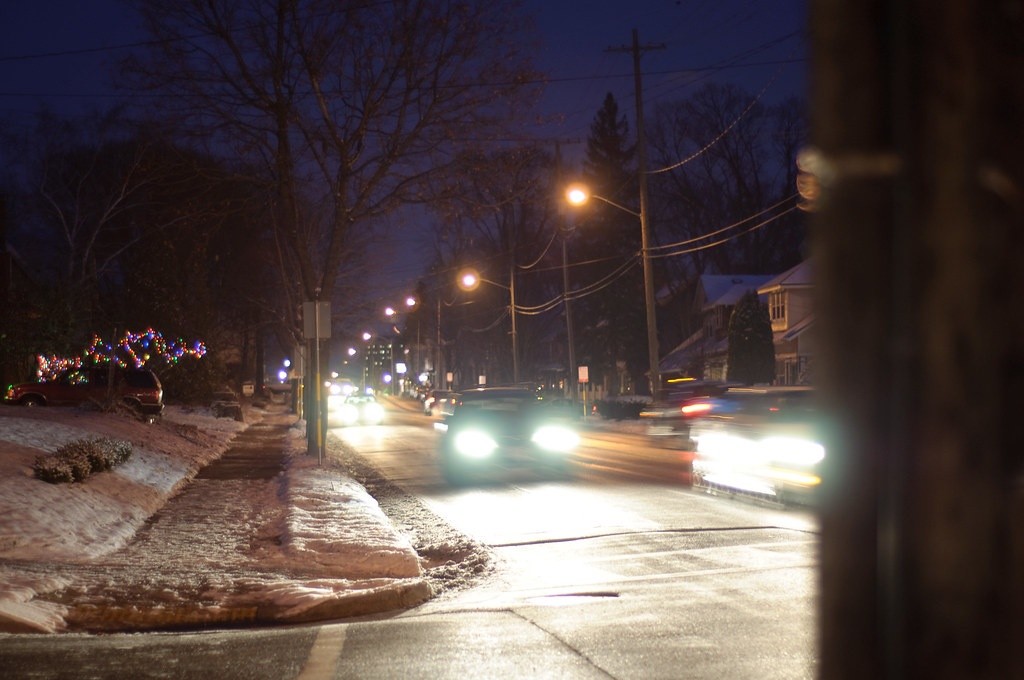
[325,379,822,502]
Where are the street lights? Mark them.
[383,307,422,380]
[458,270,520,384]
[562,182,661,398]
[406,298,442,390]
[362,332,395,396]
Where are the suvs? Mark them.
[4,366,165,415]
